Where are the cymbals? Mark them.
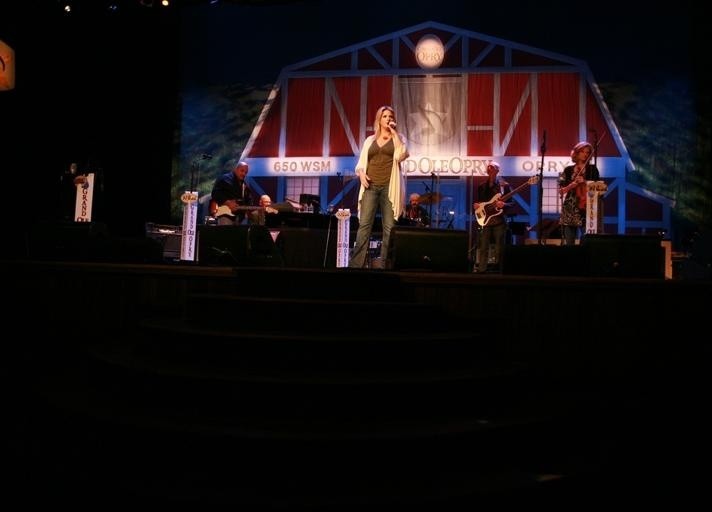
[418,192,443,205]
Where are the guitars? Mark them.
[209,200,278,221]
[475,176,539,227]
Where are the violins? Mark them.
[576,168,587,209]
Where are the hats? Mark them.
[215,206,236,217]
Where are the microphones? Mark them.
[386,119,396,132]
[421,180,433,194]
[588,127,598,133]
[541,131,546,153]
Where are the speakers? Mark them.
[576,231,663,277]
[384,226,472,271]
[197,224,249,266]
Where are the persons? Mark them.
[258,194,272,209]
[347,106,411,269]
[401,192,432,230]
[555,140,605,248]
[470,160,519,275]
[212,162,256,222]
